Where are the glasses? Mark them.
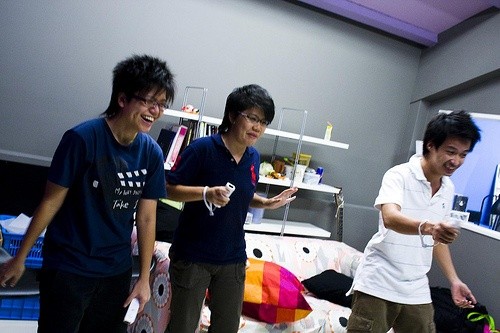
[132,93,169,111]
[237,111,271,128]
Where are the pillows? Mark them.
[204,258,313,325]
[302,268,355,308]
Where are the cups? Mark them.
[294,164,308,184]
[249,192,267,223]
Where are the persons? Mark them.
[345,109,482,332]
[165,84,298,333]
[0,55,175,332]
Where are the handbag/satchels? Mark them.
[430,287,488,333]
[467,311,500,333]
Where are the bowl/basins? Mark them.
[304,172,322,184]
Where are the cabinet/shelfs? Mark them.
[155,86,349,239]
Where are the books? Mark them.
[0,268,40,298]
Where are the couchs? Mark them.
[127,226,363,333]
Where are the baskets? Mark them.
[0,214,46,270]
